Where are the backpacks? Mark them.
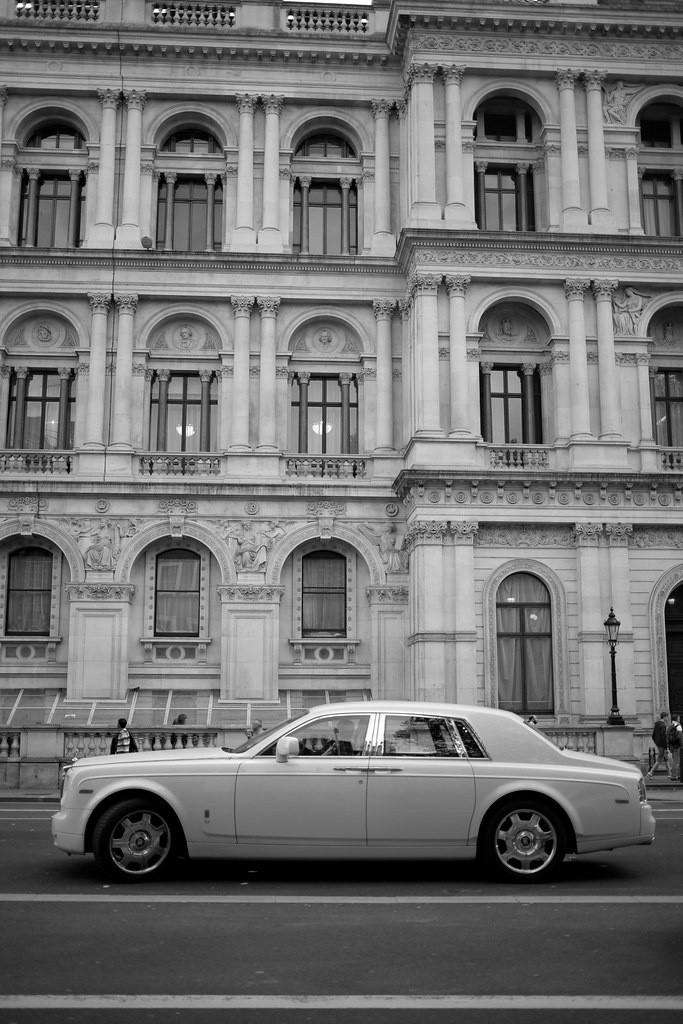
[668,724,680,750]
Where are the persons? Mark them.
[251,718,264,737]
[113,718,131,755]
[662,714,683,780]
[244,727,253,740]
[645,713,671,779]
[322,721,355,756]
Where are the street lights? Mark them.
[603,605,626,726]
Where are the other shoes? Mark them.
[667,776,671,781]
[646,773,654,781]
[670,777,680,783]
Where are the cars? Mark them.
[51,698,658,885]
